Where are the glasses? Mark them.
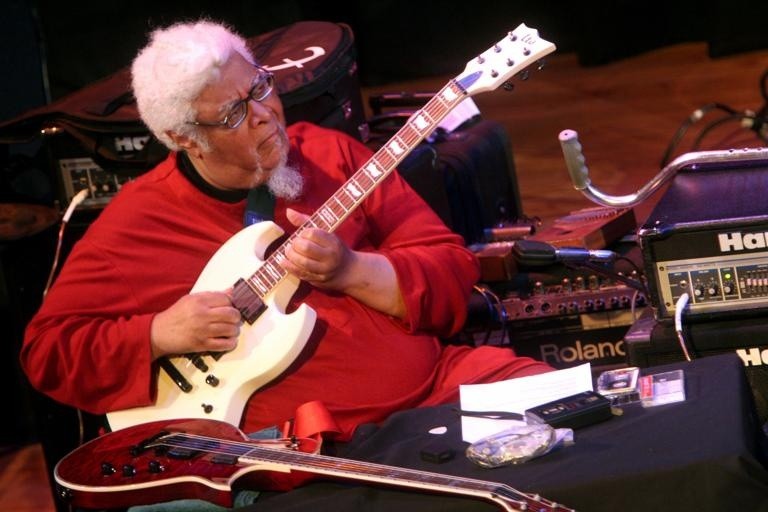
[183,66,275,128]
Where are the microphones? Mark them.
[511,240,618,265]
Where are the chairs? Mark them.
[40,181,506,510]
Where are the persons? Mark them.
[17,16,558,444]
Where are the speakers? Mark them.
[624,302,768,436]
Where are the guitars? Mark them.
[53,418,576,512]
[105,21,558,432]
